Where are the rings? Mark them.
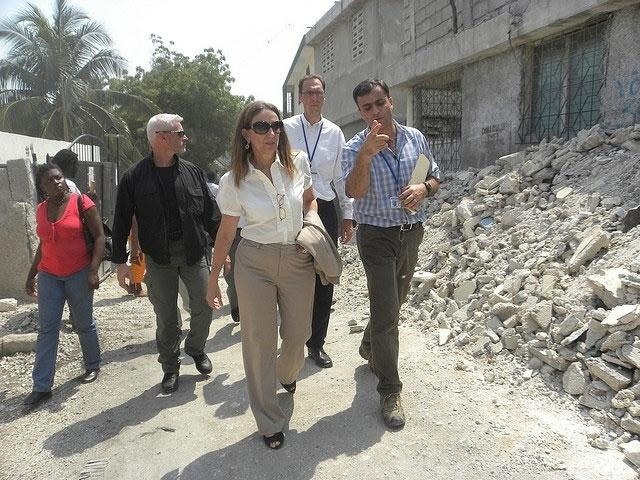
[413,198,418,203]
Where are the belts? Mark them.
[389,222,422,231]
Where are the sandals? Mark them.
[264,432,284,449]
[280,380,296,393]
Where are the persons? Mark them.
[52,149,99,202]
[205,99,318,450]
[281,73,354,368]
[110,112,242,394]
[21,163,107,406]
[340,78,442,430]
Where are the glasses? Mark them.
[156,131,185,137]
[301,91,323,97]
[250,120,284,134]
[277,194,286,220]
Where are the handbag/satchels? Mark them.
[83,220,113,262]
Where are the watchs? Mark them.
[423,181,432,196]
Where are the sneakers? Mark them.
[380,392,405,428]
[359,345,374,371]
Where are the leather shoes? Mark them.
[185,348,212,374]
[24,389,51,404]
[82,369,100,383]
[232,308,240,322]
[308,346,332,368]
[162,371,179,393]
